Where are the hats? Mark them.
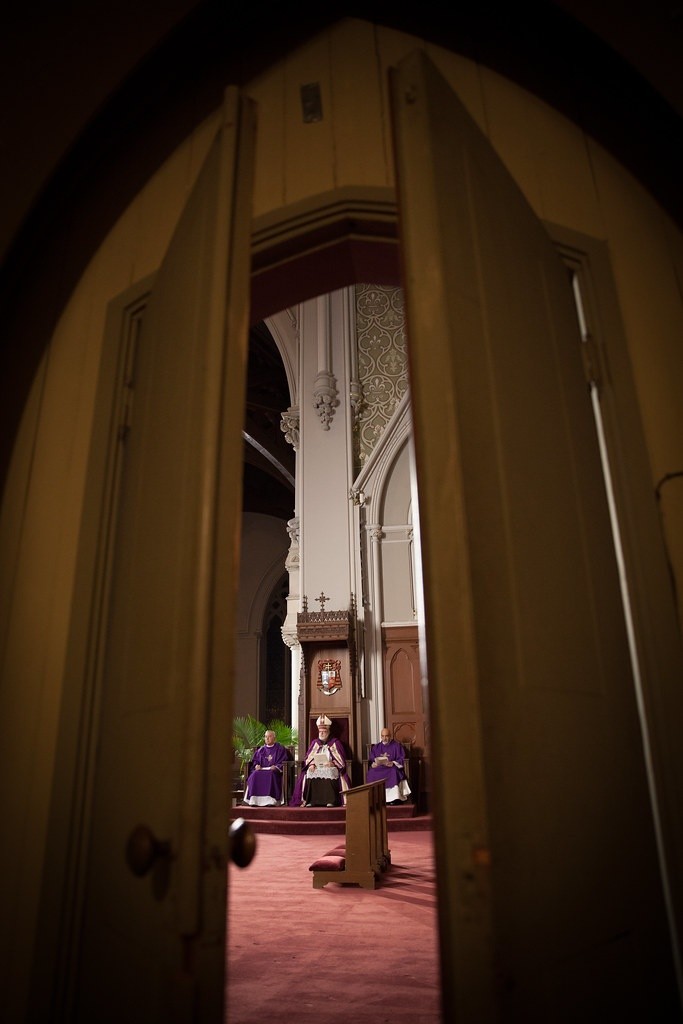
[316,715,332,731]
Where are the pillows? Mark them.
[309,855,345,872]
[323,849,346,858]
[334,844,346,849]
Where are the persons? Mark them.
[289,714,354,808]
[365,728,412,805]
[242,730,289,807]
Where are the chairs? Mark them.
[243,745,296,805]
[297,759,353,780]
[363,742,413,804]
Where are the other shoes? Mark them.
[306,804,311,807]
[391,801,396,806]
[327,804,334,808]
